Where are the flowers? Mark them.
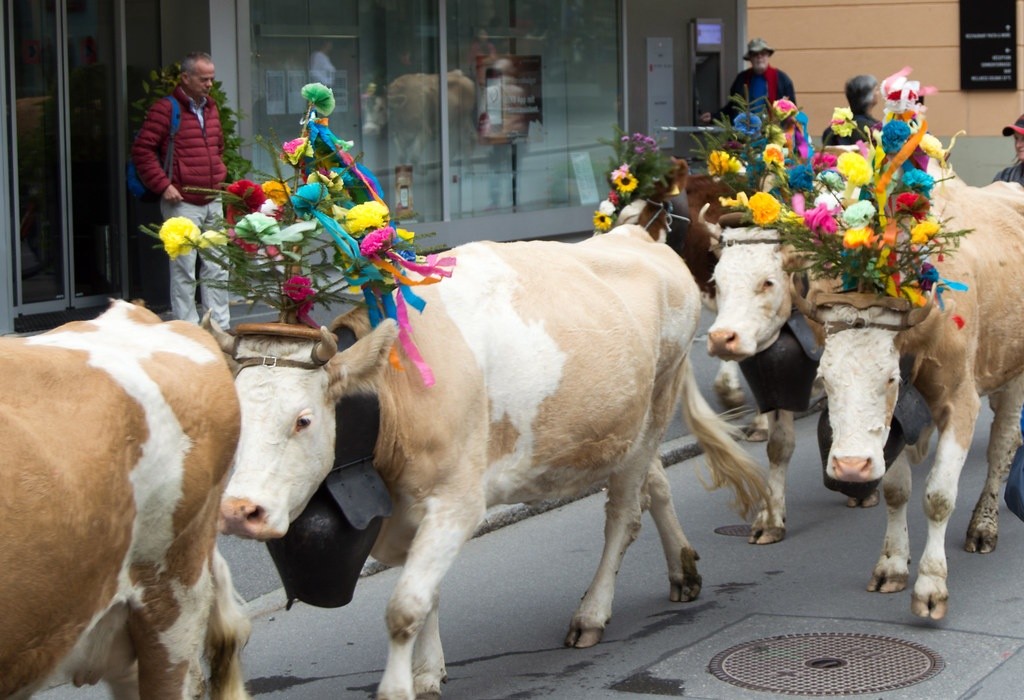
[134,79,452,393]
[591,61,976,314]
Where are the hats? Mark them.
[1003,114,1024,137]
[742,39,775,62]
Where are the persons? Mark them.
[308,34,336,86]
[699,39,797,124]
[992,114,1024,439]
[134,52,237,336]
[466,25,530,212]
[822,75,883,145]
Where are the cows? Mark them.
[359,68,479,180]
[0,156,1024,700]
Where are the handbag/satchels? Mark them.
[127,96,181,204]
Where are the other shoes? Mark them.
[224,328,236,337]
